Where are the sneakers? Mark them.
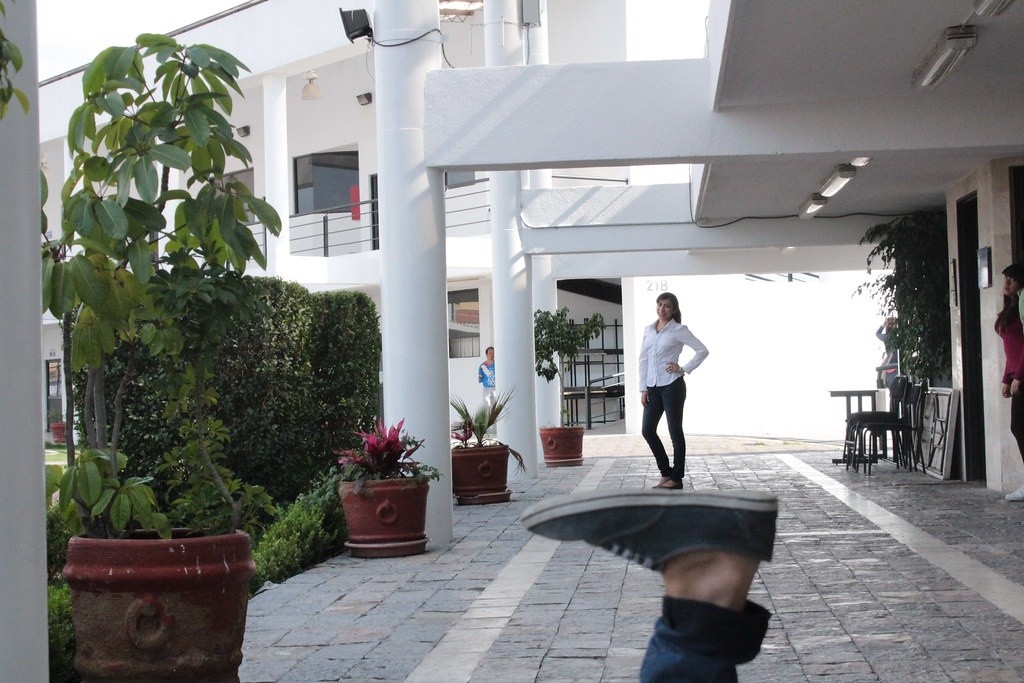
[520,488,778,576]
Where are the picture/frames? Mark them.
[948,258,958,307]
[976,246,993,290]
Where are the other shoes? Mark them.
[650,479,683,489]
[1005,486,1024,500]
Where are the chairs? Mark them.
[843,374,926,475]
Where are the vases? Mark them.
[337,478,431,559]
[451,444,514,505]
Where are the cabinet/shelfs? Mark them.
[563,318,625,430]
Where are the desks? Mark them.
[828,389,879,465]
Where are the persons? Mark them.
[640,292,709,490]
[520,488,778,683]
[479,347,495,406]
[876,317,899,388]
[994,264,1024,501]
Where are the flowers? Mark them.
[332,417,445,496]
[450,384,528,477]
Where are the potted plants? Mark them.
[533,305,606,468]
[46,404,66,443]
[39,32,283,683]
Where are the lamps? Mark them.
[910,25,977,96]
[797,192,827,220]
[356,92,373,107]
[819,163,857,198]
[973,0,1013,17]
[338,7,373,45]
[236,125,251,138]
[300,70,324,100]
